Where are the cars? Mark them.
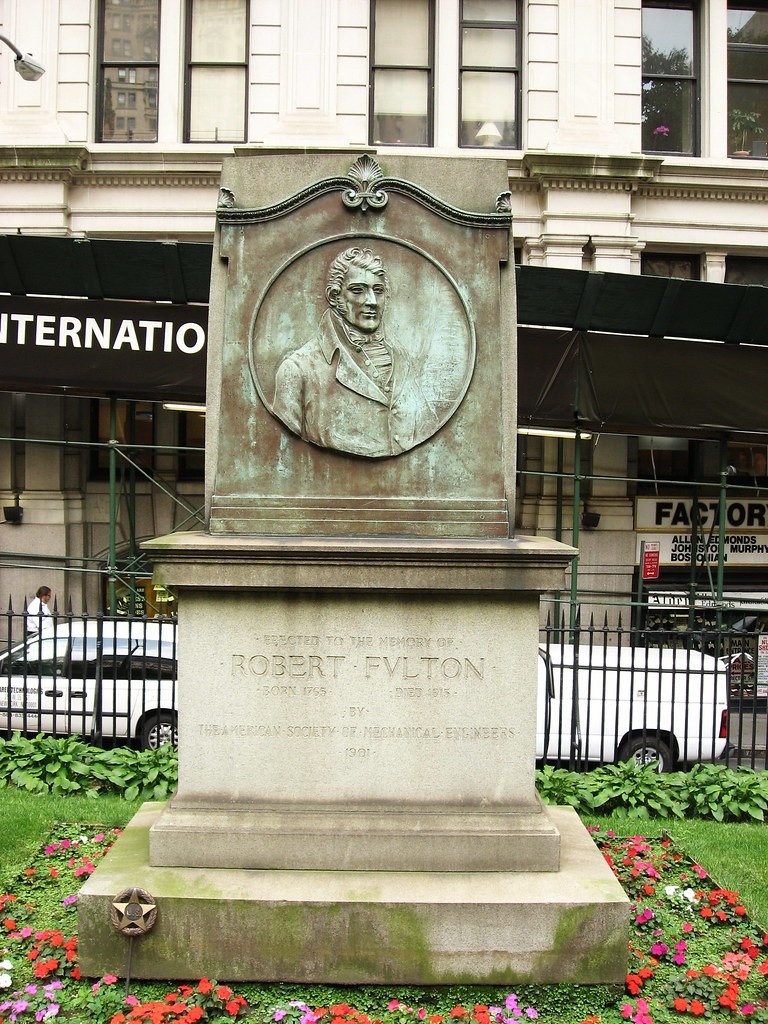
[0,621,181,754]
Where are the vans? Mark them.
[535,641,729,774]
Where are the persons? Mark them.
[271,248,438,456]
[27,586,53,637]
